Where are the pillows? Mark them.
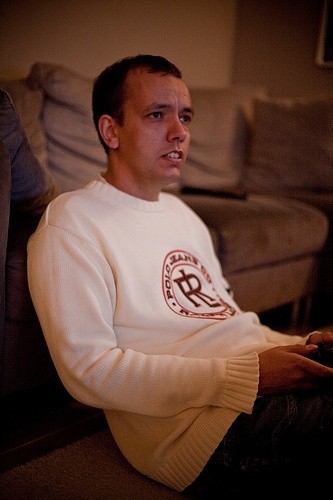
[0,62,333,215]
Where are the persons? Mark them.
[27,55,333,500]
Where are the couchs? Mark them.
[1,60,333,327]
